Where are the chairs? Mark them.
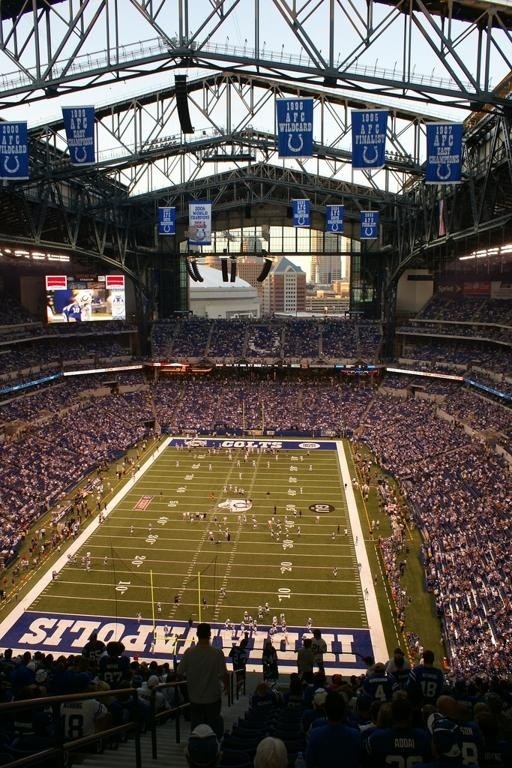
[0,669,512,768]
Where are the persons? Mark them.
[0,292,511,768]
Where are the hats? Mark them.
[428,712,465,757]
[147,675,159,687]
[190,723,216,740]
[35,668,49,683]
